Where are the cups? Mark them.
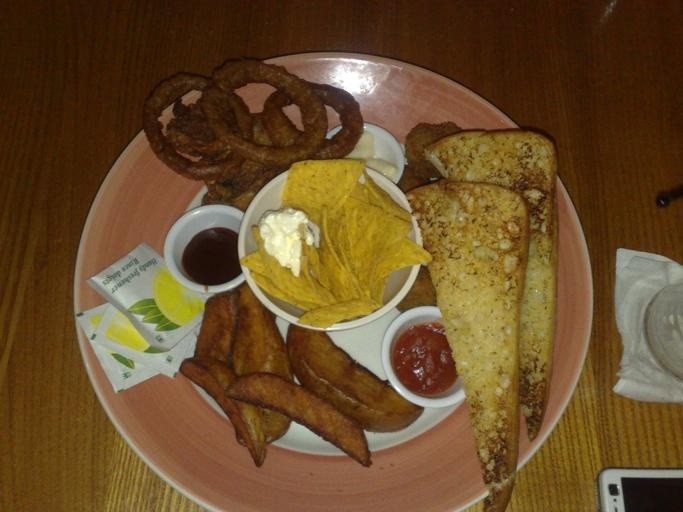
[642,281,683,381]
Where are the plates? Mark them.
[70,49,596,512]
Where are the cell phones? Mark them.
[597,468,683,512]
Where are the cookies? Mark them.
[404,129,558,512]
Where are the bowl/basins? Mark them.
[163,203,246,293]
[238,163,424,331]
[323,121,406,187]
[379,304,467,408]
